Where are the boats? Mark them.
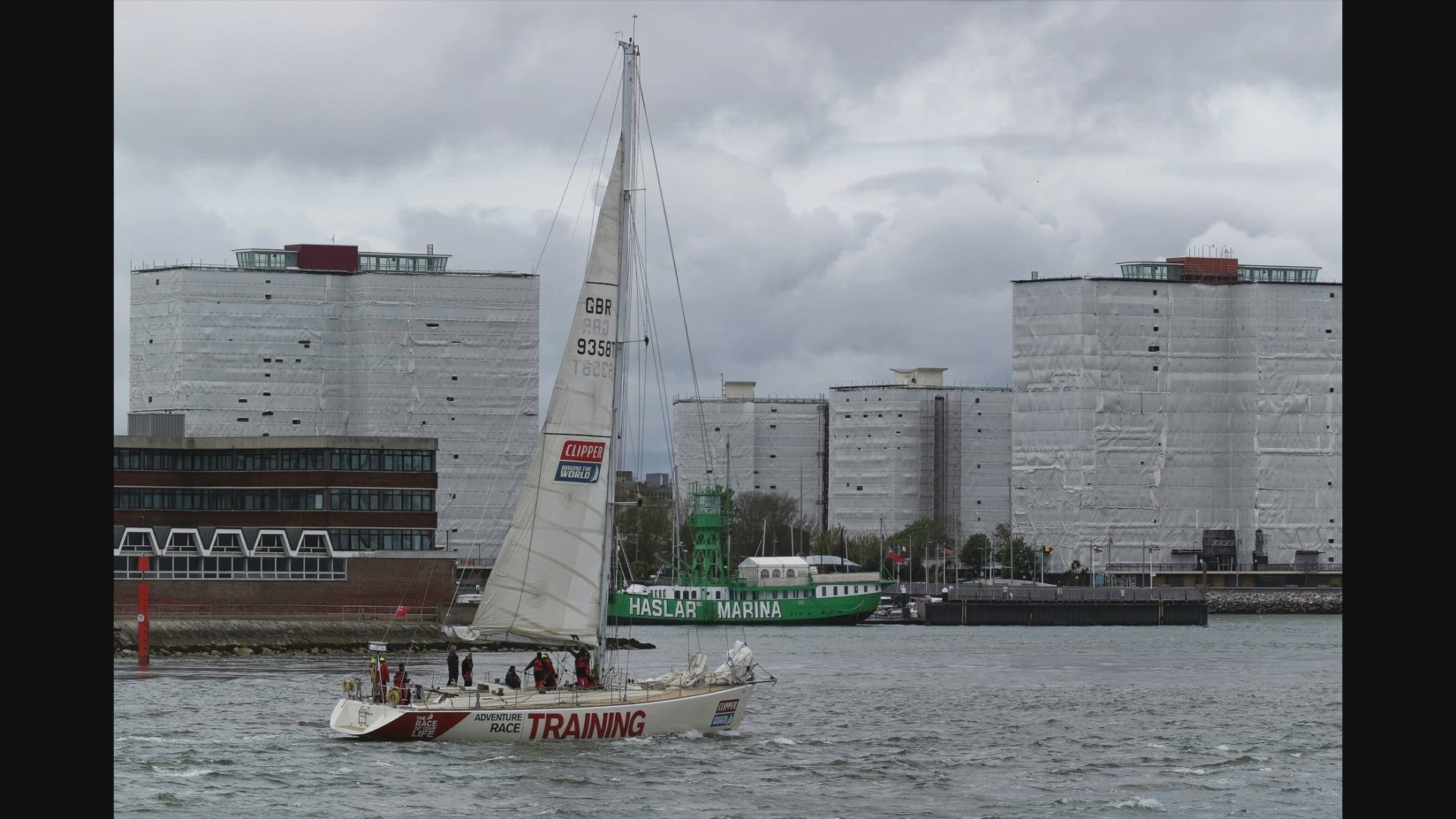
[607,433,900,626]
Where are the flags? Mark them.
[900,545,902,552]
[1042,545,1053,552]
[887,552,905,563]
[902,545,910,552]
[946,546,953,554]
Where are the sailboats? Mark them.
[325,8,780,746]
[867,517,1058,619]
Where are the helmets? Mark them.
[541,655,547,658]
[370,659,377,663]
[380,657,385,661]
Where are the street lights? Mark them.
[1192,541,1198,571]
[1147,548,1153,591]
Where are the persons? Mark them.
[447,646,459,687]
[564,643,591,690]
[369,652,390,703]
[505,665,521,690]
[393,663,411,683]
[522,650,558,691]
[462,653,473,686]
